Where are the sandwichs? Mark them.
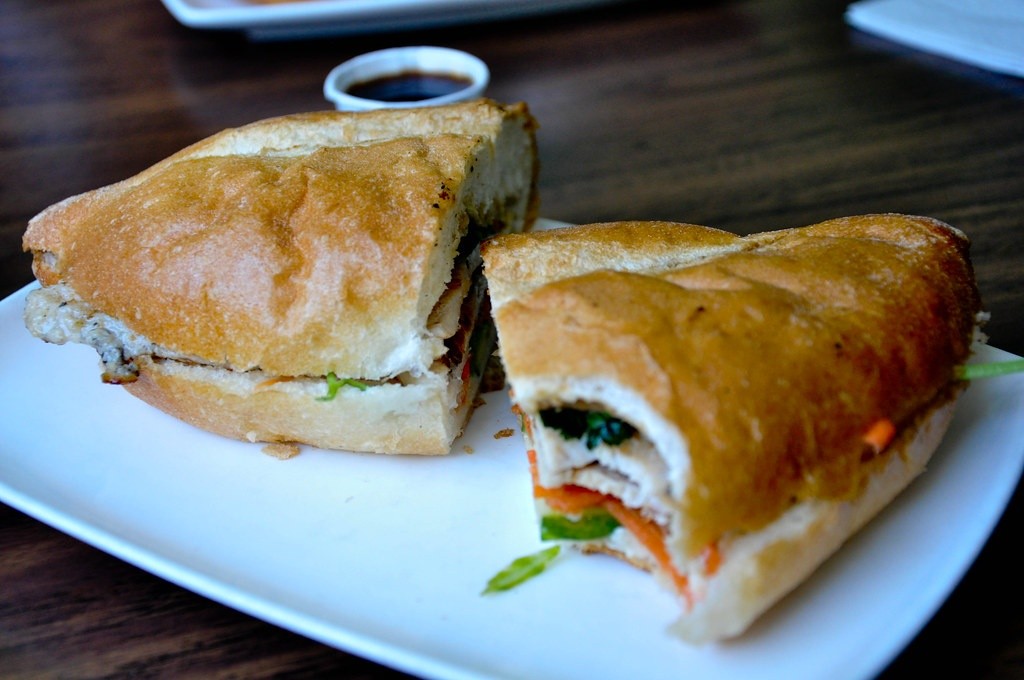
[479,213,1024,648]
[21,99,541,457]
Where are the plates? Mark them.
[0,215,1024,680]
[845,0,1024,84]
[159,0,591,38]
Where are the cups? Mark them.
[321,44,490,118]
